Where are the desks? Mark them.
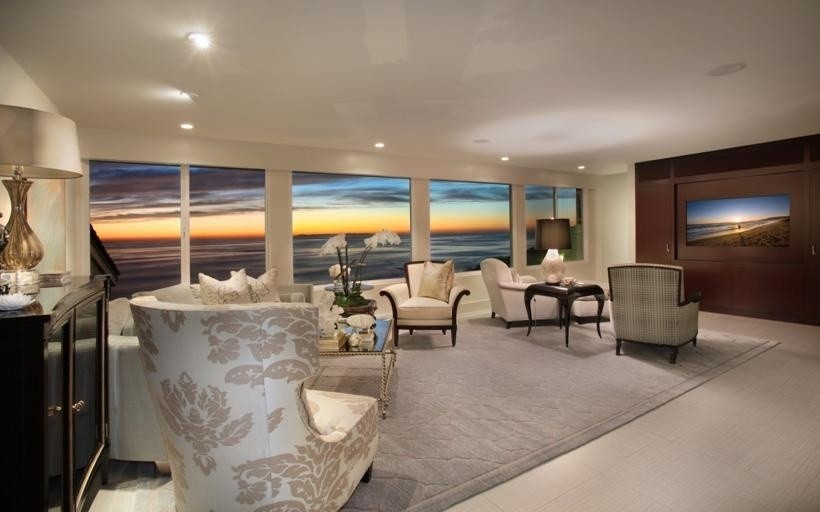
[324,283,375,310]
[524,280,605,347]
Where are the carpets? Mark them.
[296,305,782,512]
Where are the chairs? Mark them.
[478,255,565,330]
[379,258,472,346]
[605,256,704,363]
[129,292,382,512]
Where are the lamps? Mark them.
[0,100,85,298]
[534,216,572,285]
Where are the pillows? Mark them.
[418,259,455,304]
[122,314,137,337]
[197,269,252,303]
[230,267,283,302]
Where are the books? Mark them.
[40,270,71,287]
[319,329,350,352]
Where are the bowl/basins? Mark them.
[560,279,576,287]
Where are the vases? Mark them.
[334,279,344,289]
[341,299,378,321]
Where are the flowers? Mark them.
[328,264,351,278]
[314,223,401,310]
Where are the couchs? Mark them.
[569,278,610,325]
[106,275,317,475]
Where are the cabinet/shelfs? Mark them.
[1,272,110,512]
